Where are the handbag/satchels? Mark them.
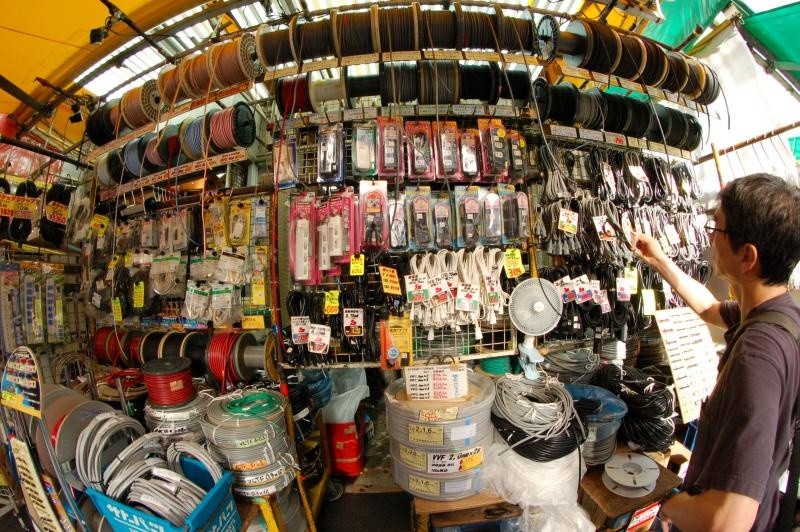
[319,367,370,424]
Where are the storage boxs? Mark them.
[325,402,366,478]
[86,454,242,532]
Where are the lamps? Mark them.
[70,102,83,123]
[90,11,122,45]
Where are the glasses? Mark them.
[704,220,731,235]
[602,218,642,261]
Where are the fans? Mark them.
[508,278,563,380]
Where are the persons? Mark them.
[630,173,800,532]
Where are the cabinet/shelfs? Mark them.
[413,492,522,532]
[578,447,683,532]
